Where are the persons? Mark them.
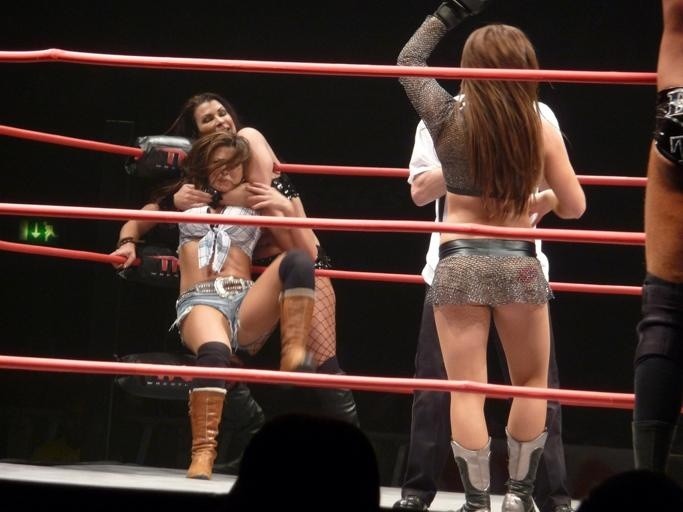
[224,412,381,512]
[629,2,683,471]
[388,94,572,510]
[156,90,362,476]
[397,2,591,511]
[111,130,315,481]
[577,468,681,511]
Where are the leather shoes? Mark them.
[393,495,428,511]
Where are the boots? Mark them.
[213,381,267,475]
[187,387,227,479]
[502,427,548,511]
[278,287,318,372]
[447,436,492,512]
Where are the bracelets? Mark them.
[208,190,223,209]
[158,192,177,210]
[117,236,142,248]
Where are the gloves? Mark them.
[433,0,489,33]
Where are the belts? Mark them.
[175,275,254,312]
